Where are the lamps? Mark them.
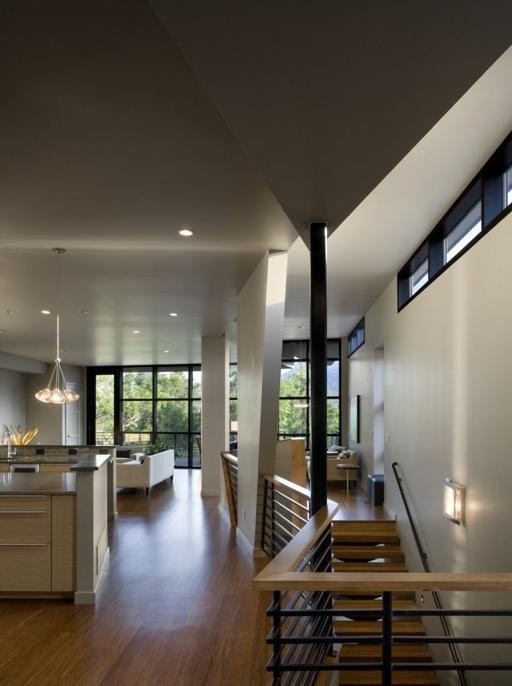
[32,252,80,404]
[440,480,466,526]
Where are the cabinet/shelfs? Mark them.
[0,494,76,601]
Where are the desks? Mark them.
[336,464,359,495]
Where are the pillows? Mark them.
[328,445,350,459]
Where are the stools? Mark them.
[366,473,384,507]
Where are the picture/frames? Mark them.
[348,394,360,443]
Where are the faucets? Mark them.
[2,430,17,460]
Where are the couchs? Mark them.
[304,445,358,488]
[115,447,175,497]
